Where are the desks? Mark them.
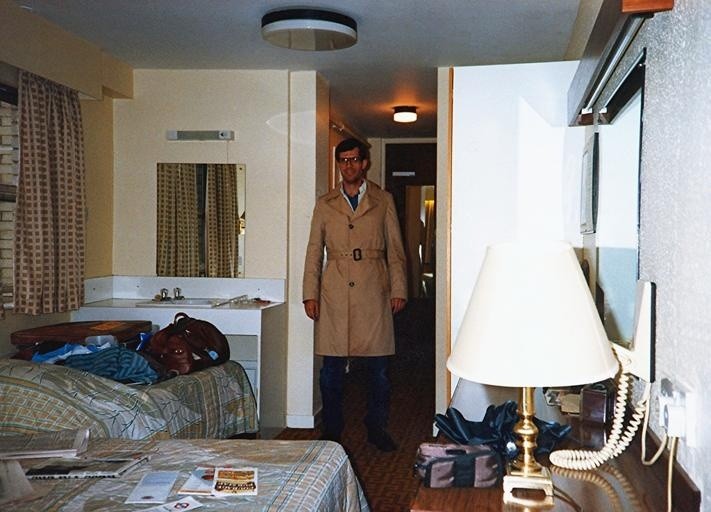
[408,376,700,512]
[72,297,290,441]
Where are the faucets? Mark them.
[173,287,185,300]
[160,288,171,302]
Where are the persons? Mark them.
[299,140,408,450]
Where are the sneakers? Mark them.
[366,426,396,452]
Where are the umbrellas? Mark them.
[433,402,571,458]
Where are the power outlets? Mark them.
[658,381,695,448]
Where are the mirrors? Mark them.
[593,45,650,350]
[156,162,247,279]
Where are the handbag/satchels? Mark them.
[142,312,231,375]
[412,441,501,491]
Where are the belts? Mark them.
[325,248,385,261]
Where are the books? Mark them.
[178,464,260,497]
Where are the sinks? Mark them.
[136,297,230,307]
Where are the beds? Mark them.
[0,439,374,510]
[0,358,260,440]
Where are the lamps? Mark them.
[447,239,621,507]
[10,318,154,365]
[260,6,359,53]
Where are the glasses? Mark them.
[340,156,360,162]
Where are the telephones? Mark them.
[549,281,656,468]
